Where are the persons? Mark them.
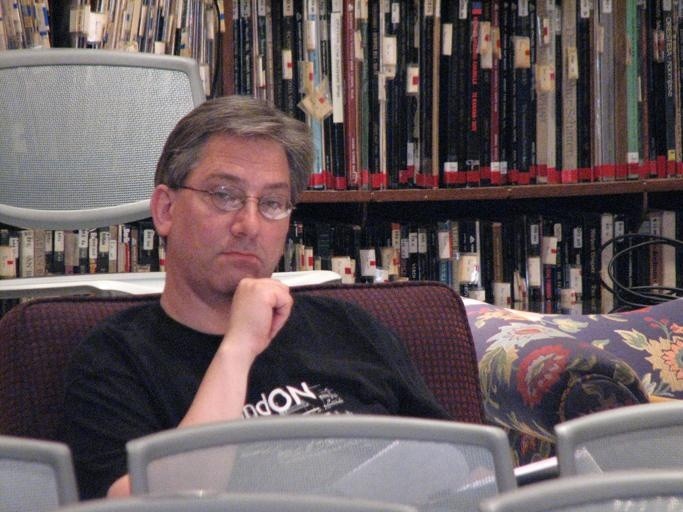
[47,91,464,500]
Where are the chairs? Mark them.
[0,48,683,512]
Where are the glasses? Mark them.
[182,183,295,221]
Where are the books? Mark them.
[452,446,602,497]
[0,0,681,330]
